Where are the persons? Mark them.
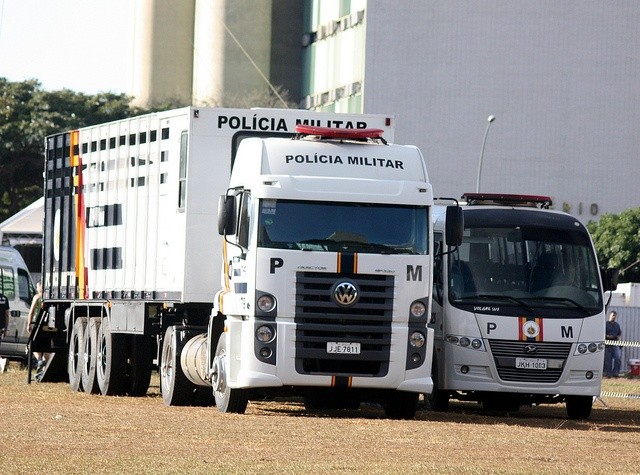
[26,279,49,373]
[605,311,622,378]
[0,292,10,374]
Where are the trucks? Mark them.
[42,106,464,420]
[431,194,617,419]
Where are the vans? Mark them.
[1,245,38,369]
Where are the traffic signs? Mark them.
[477,116,495,194]
